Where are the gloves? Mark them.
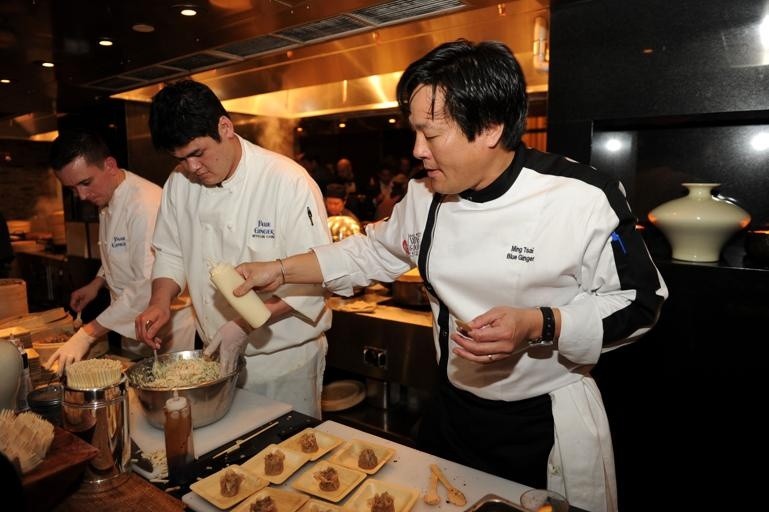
[204,317,254,377]
[45,328,94,378]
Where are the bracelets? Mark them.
[275,259,286,285]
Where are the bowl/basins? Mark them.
[389,280,429,306]
[26,385,61,416]
[126,347,248,430]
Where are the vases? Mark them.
[646,182,753,262]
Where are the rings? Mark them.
[488,354,493,362]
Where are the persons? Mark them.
[233,38,670,512]
[301,159,421,242]
[44,129,198,377]
[134,78,333,421]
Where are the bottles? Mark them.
[162,382,195,477]
[202,254,273,330]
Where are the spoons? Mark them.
[431,462,467,505]
[422,475,438,506]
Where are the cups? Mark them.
[519,488,570,512]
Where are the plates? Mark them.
[188,425,420,512]
[319,378,366,413]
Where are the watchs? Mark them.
[528,306,555,349]
